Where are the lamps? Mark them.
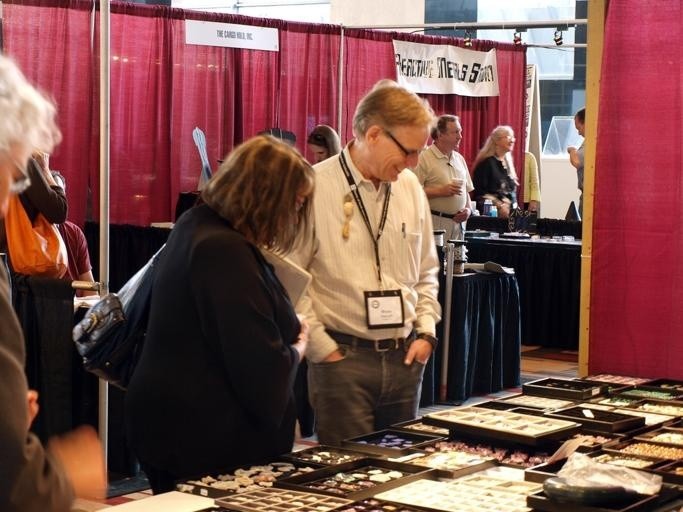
[553,30,563,47]
[512,31,523,47]
[463,30,473,48]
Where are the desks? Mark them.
[0,203,584,406]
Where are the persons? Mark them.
[473,126,518,216]
[414,114,475,254]
[524,152,540,216]
[290,80,441,444]
[568,108,586,220]
[130,135,316,495]
[296,125,342,442]
[1,55,108,510]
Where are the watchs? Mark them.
[417,334,440,352]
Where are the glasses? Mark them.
[497,135,517,142]
[385,131,419,157]
[3,152,31,193]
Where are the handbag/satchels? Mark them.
[73,293,145,392]
[5,195,69,280]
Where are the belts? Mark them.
[431,211,454,219]
[329,332,412,351]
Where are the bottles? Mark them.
[483,199,498,217]
